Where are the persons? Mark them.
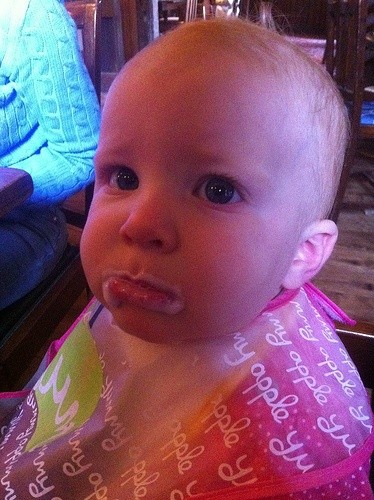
[335,0,374,97]
[0,0,107,309]
[0,17,374,500]
